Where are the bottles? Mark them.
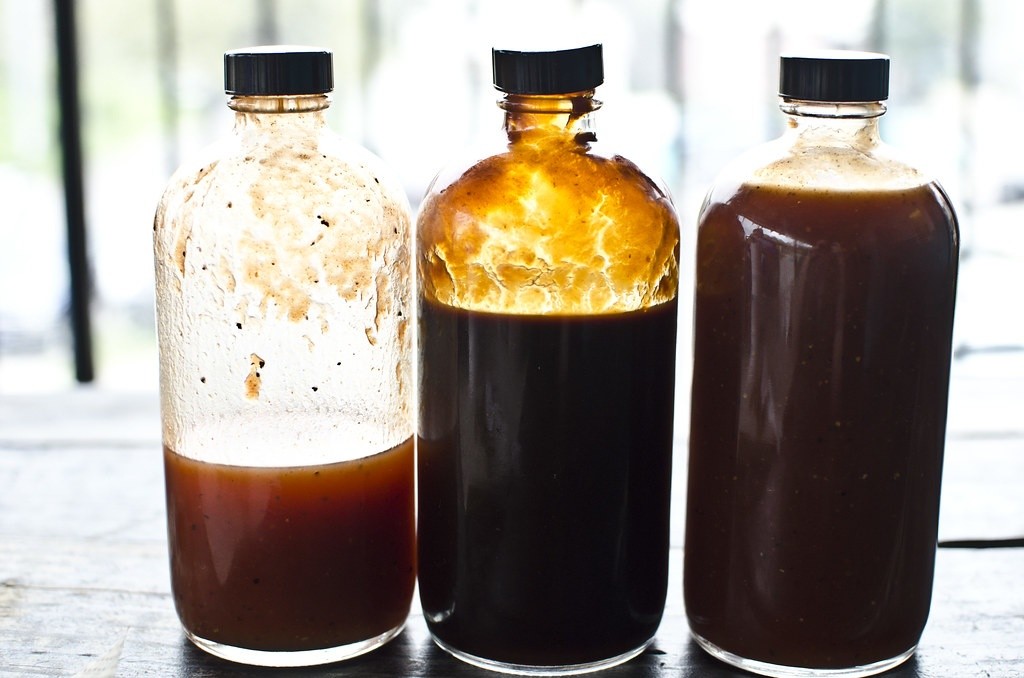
[150,45,421,668]
[680,49,960,678]
[415,42,681,677]
